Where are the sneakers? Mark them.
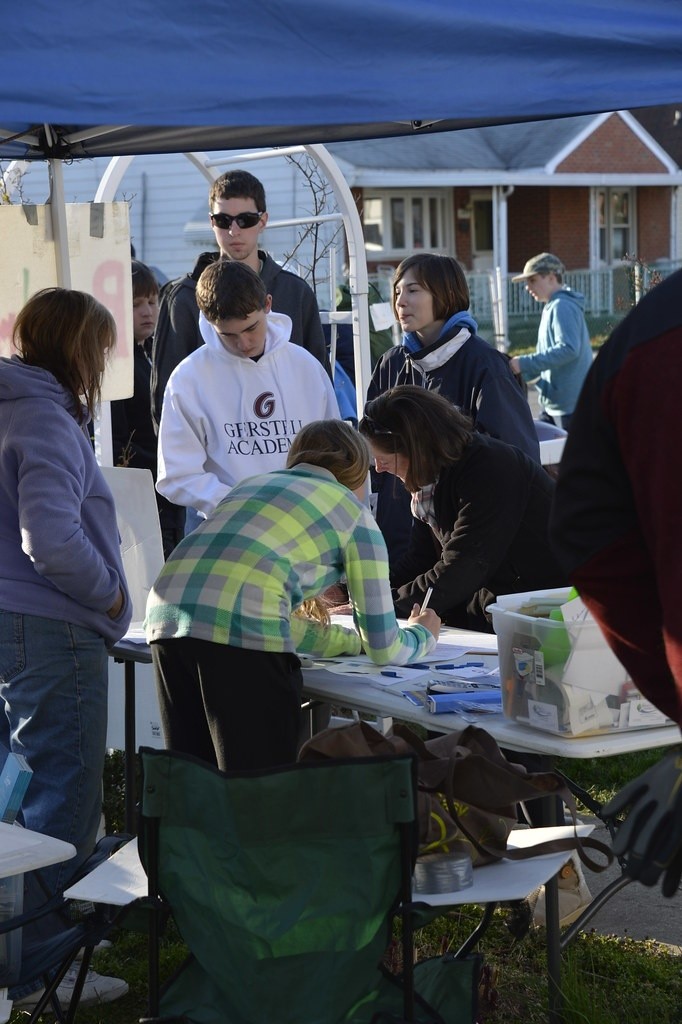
[14,959,129,1013]
[76,940,113,958]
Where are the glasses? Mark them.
[209,213,261,230]
[362,401,400,443]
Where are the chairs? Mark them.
[131,742,479,1024]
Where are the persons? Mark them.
[508,254,594,432]
[358,251,542,544]
[2,285,133,1004]
[102,241,184,563]
[143,419,440,785]
[155,256,345,544]
[321,385,573,629]
[155,168,319,576]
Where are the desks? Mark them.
[109,611,682,1024]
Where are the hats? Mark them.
[512,253,565,282]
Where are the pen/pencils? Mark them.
[466,651,500,655]
[400,663,430,669]
[418,586,433,616]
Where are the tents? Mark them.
[2,0,682,298]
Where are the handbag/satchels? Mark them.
[296,717,622,873]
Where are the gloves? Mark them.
[632,846,682,898]
[601,744,682,869]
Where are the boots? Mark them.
[529,814,596,932]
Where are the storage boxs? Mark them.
[484,585,677,739]
